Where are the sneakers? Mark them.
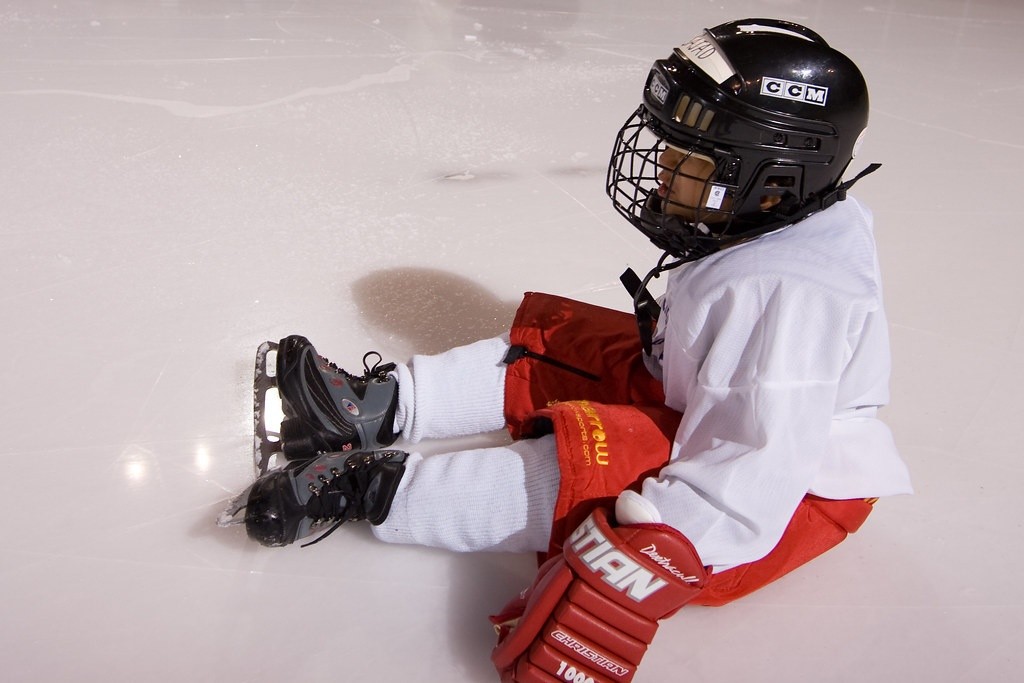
[252,335,400,479]
[215,450,408,548]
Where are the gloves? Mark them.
[488,507,714,683]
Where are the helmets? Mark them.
[640,18,869,255]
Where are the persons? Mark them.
[216,17,913,683]
